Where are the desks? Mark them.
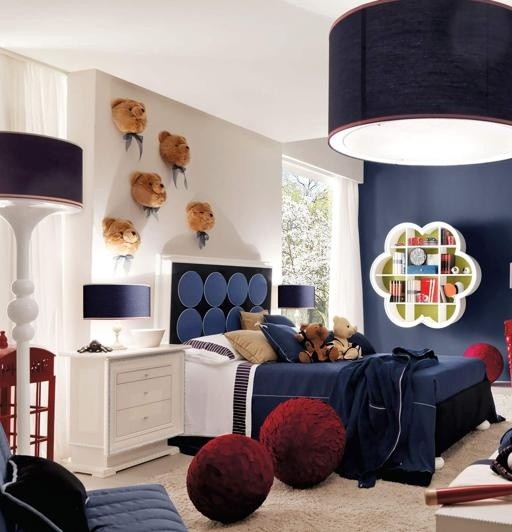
[434,448,512,532]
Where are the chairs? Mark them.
[1,425,187,532]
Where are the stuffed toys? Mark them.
[110,96,147,162]
[294,322,339,363]
[187,201,216,249]
[100,215,142,282]
[128,170,166,220]
[331,315,362,360]
[158,130,191,192]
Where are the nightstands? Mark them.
[70,347,185,479]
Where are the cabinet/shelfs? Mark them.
[158,256,506,487]
[370,221,482,329]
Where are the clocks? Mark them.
[409,248,426,265]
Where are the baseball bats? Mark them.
[424,484,512,505]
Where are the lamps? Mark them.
[0,130,84,455]
[83,285,150,350]
[279,285,316,330]
[327,0,512,166]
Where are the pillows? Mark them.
[183,310,376,364]
[2,455,90,532]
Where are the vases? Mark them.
[131,329,165,348]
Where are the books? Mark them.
[388,229,458,304]
